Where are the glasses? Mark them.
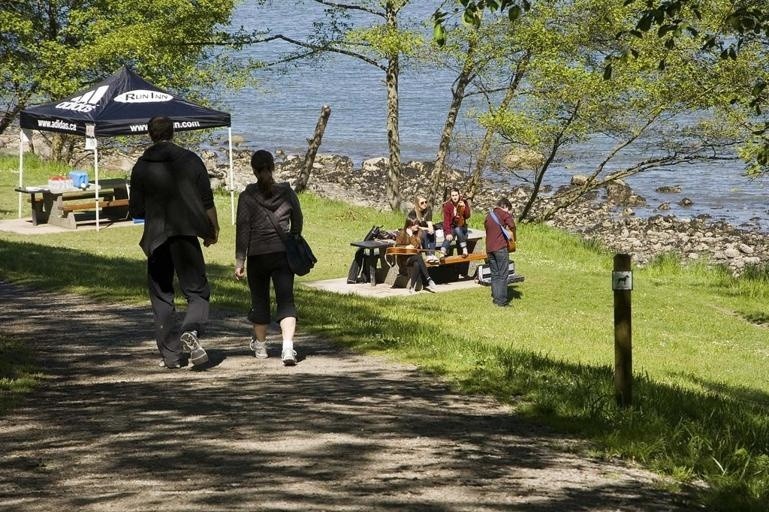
[421,201,427,204]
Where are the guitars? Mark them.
[386,247,443,255]
[505,229,516,252]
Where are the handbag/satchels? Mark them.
[285,231,317,276]
[507,238,516,252]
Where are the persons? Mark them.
[483,198,517,306]
[441,188,470,259]
[233,150,313,365]
[397,216,439,293]
[128,113,220,370]
[406,195,440,265]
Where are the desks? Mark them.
[350,227,487,287]
[14,178,130,227]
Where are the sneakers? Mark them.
[281,348,297,366]
[159,359,180,369]
[428,282,441,292]
[494,301,514,307]
[409,286,416,295]
[440,250,448,256]
[180,331,208,365]
[462,248,468,258]
[426,255,439,263]
[250,339,268,359]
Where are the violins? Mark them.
[452,193,465,227]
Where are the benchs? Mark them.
[27,188,130,230]
[423,250,487,291]
